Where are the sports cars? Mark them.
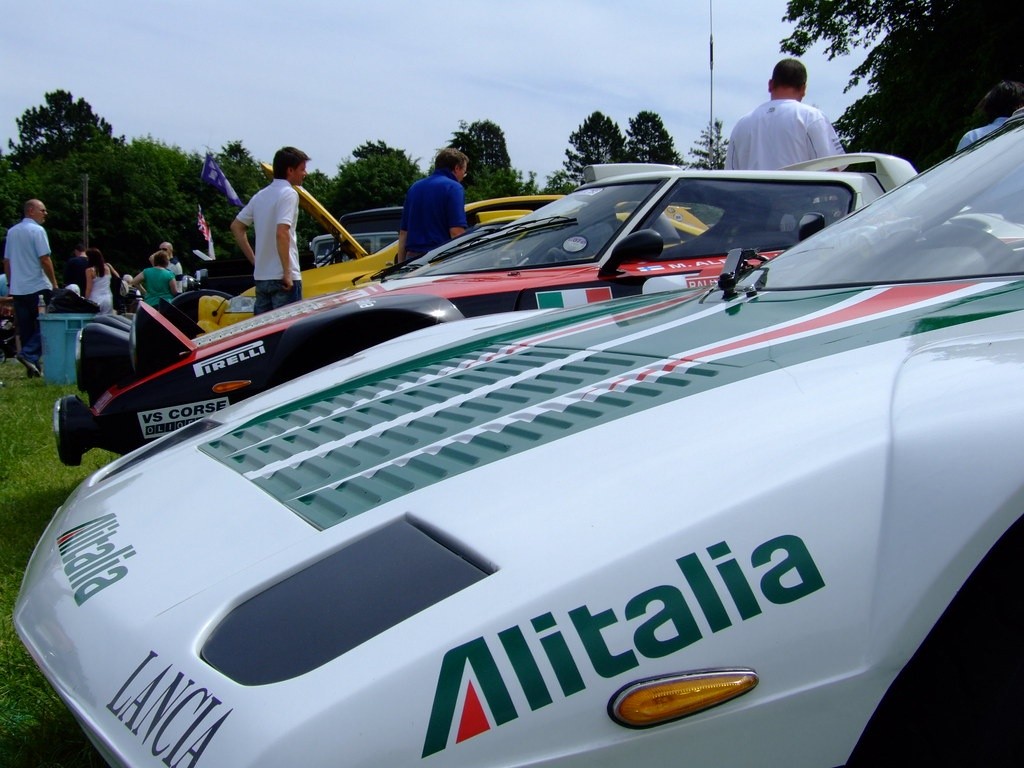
[51,153,1024,468]
[195,194,712,333]
[13,107,1023,768]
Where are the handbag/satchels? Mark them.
[110,273,122,298]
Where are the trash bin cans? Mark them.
[36,313,94,384]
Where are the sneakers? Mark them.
[15,354,43,377]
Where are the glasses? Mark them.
[159,248,170,251]
[462,168,467,178]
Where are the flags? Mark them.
[201,152,244,208]
[197,204,216,260]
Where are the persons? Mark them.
[63,242,184,315]
[724,58,846,170]
[398,148,469,263]
[4,199,59,378]
[229,146,311,316]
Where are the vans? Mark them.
[309,207,406,268]
